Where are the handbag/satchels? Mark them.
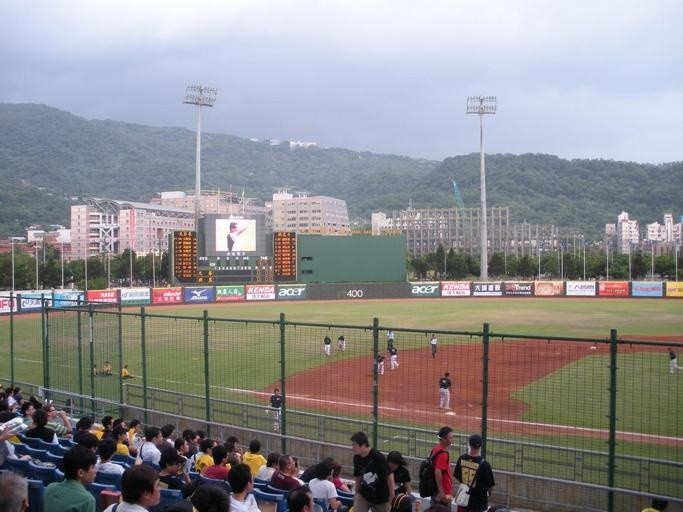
[451,481,471,509]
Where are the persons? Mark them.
[388,344,399,370]
[430,334,437,357]
[336,336,345,351]
[430,425,453,511]
[101,360,112,376]
[1,385,421,510]
[386,329,394,352]
[438,372,453,410]
[120,364,136,379]
[640,496,668,511]
[93,364,106,377]
[453,434,495,511]
[668,347,682,374]
[323,336,331,357]
[377,351,386,376]
[227,222,246,251]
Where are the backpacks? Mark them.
[417,449,449,498]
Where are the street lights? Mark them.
[181,82,219,284]
[464,94,499,284]
[10,237,27,290]
[85,253,97,290]
[34,245,41,290]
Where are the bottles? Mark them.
[347,481,353,491]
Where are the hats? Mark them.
[469,434,483,448]
